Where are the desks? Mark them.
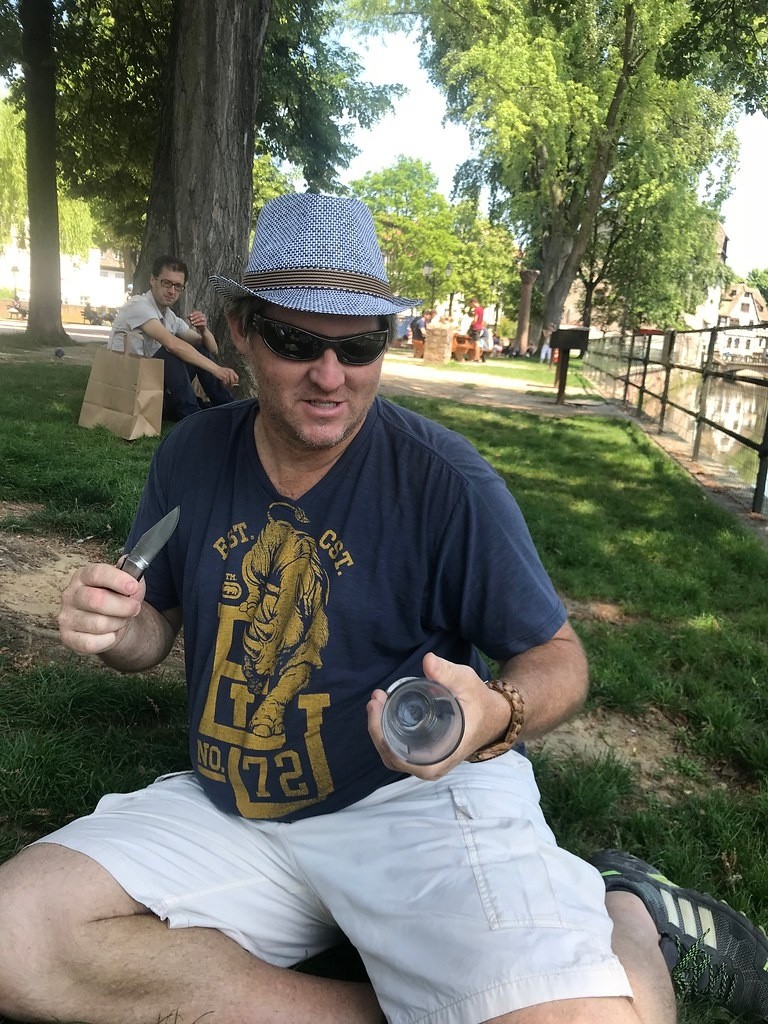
[454,332,468,340]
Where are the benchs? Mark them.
[81,311,93,324]
[7,305,28,319]
[414,340,472,360]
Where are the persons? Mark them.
[12,296,26,320]
[416,309,437,343]
[539,323,556,364]
[108,255,240,420]
[0,193,768,1024]
[469,296,503,363]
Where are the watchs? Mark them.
[465,677,525,763]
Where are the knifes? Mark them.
[120,505,181,584]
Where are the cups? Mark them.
[381,675,465,766]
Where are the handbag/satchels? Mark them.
[77,331,164,440]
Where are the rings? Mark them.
[202,317,204,320]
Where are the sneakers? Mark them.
[587,848,768,1024]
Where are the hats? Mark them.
[209,193,421,316]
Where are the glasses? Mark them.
[251,313,389,366]
[155,275,185,292]
